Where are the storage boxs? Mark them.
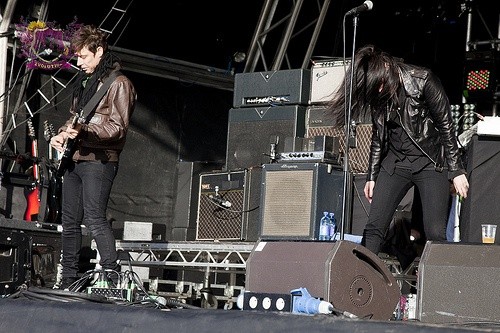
[195,167,249,241]
[305,106,373,173]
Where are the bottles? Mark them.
[319,212,330,240]
[329,213,336,240]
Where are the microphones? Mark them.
[345,0,374,16]
[208,195,231,208]
[25,48,52,63]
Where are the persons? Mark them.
[324,46,468,264]
[48,24,137,293]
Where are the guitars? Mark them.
[54,109,87,179]
[43,119,63,224]
[22,119,53,224]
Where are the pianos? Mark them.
[457,116,500,148]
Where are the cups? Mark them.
[481,224,497,243]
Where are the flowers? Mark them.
[14,16,86,70]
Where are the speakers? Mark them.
[416,241,500,324]
[246,242,400,323]
[195,58,373,242]
[121,221,166,280]
